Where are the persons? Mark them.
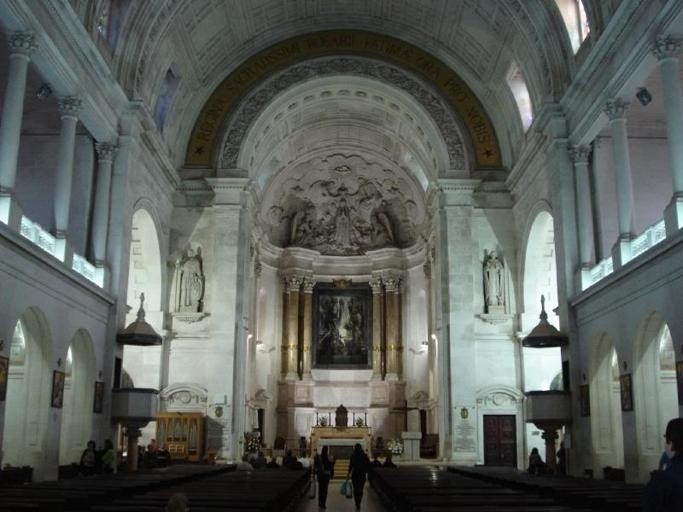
[528,448,540,474]
[312,445,334,510]
[236,449,298,473]
[173,248,204,313]
[80,438,170,476]
[383,457,397,468]
[288,178,395,255]
[554,440,566,475]
[370,455,384,468]
[640,418,682,511]
[481,250,505,308]
[346,442,370,512]
[163,493,191,512]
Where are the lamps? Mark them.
[111,388,159,475]
[116,292,164,347]
[519,294,571,353]
[522,389,575,477]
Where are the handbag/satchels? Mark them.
[340,481,352,498]
[307,478,316,499]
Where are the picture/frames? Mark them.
[578,361,683,417]
[0,356,105,414]
[312,282,373,370]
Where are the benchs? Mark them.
[0,465,312,512]
[366,465,659,512]
